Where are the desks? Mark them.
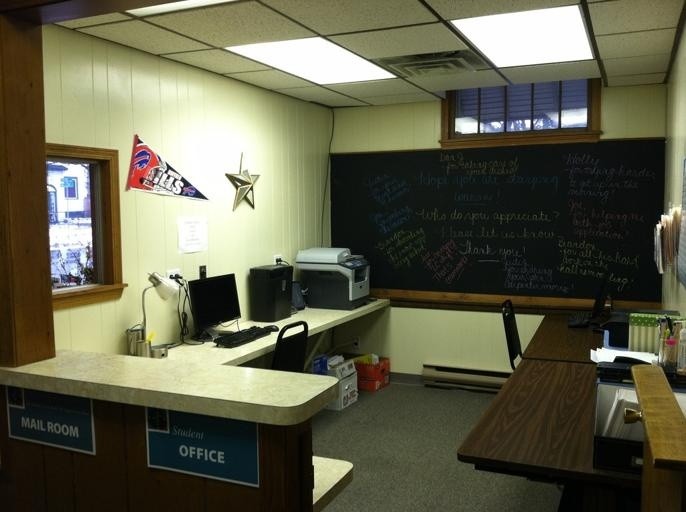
[163,296,391,367]
[521,309,685,366]
[622,363,686,512]
[456,356,686,511]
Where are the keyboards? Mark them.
[214,326,271,348]
[566,310,592,329]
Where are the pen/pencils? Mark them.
[657,315,682,365]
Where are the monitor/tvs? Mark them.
[590,276,616,325]
[184,272,241,342]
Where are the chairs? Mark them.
[268,319,310,372]
[500,297,524,370]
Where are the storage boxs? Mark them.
[306,354,360,413]
[353,355,393,393]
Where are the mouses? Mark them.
[264,325,279,332]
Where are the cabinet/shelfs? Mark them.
[0,351,355,512]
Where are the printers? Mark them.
[295,245,371,311]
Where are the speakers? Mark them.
[249,263,294,322]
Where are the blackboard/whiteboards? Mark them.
[328,135,667,310]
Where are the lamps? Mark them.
[141,270,179,343]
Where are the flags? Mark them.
[127,134,209,201]
